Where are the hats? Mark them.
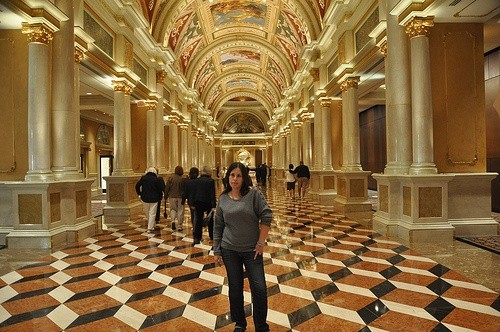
[146,168,157,176]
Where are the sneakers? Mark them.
[147,230,156,234]
[234,319,247,332]
[194,238,200,244]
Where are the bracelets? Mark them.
[256,240,266,248]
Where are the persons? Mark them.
[134,167,163,233]
[190,165,217,247]
[154,168,166,224]
[180,167,200,230]
[286,164,297,199]
[254,162,271,187]
[216,162,227,188]
[289,161,310,199]
[163,165,189,231]
[213,161,273,332]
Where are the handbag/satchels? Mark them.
[164,213,167,218]
[200,211,213,227]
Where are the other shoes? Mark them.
[178,228,183,231]
[291,195,295,200]
[299,196,304,199]
[172,221,176,230]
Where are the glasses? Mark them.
[229,173,244,177]
